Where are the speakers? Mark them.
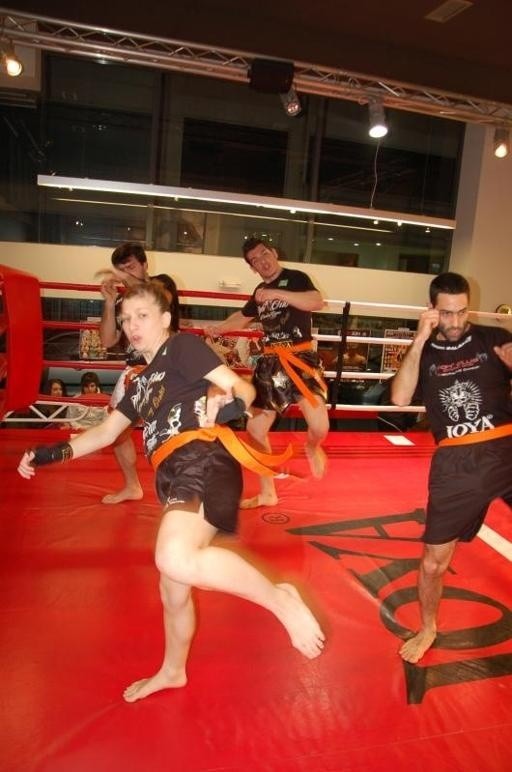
[247,58,294,94]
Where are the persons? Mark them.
[17,276,329,705]
[332,343,366,405]
[154,210,203,254]
[97,240,183,505]
[378,384,433,432]
[65,372,113,430]
[204,237,332,511]
[29,377,71,431]
[388,270,512,667]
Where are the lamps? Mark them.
[362,95,388,138]
[35,171,459,234]
[0,41,22,77]
[277,79,302,117]
[491,122,509,160]
